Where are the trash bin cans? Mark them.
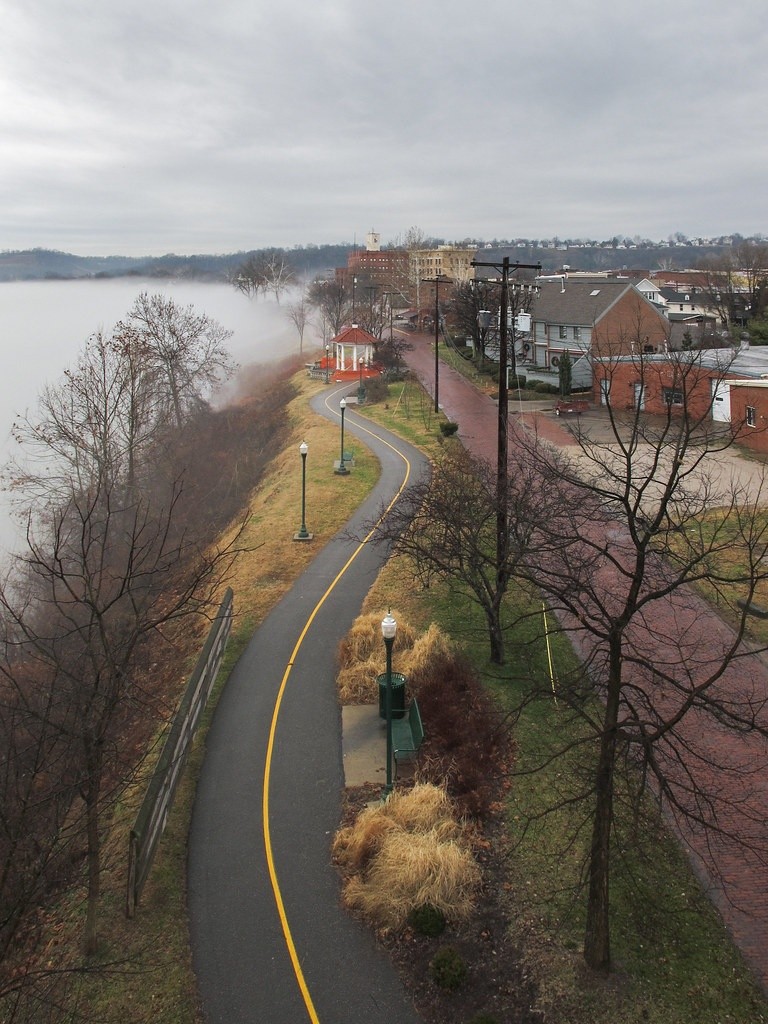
[376,672,408,719]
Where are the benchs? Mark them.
[391,697,424,781]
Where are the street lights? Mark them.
[298,439,310,538]
[381,602,397,801]
[359,357,364,395]
[324,344,330,384]
[339,397,346,471]
